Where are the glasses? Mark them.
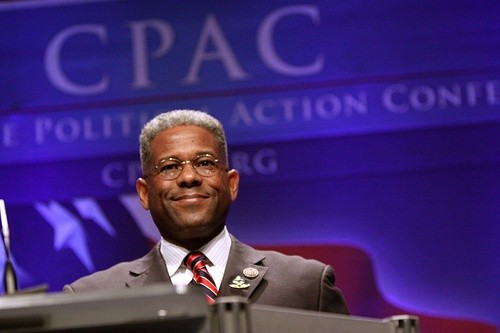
[143,154,228,181]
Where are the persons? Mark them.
[61,110,351,317]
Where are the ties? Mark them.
[182,251,219,307]
